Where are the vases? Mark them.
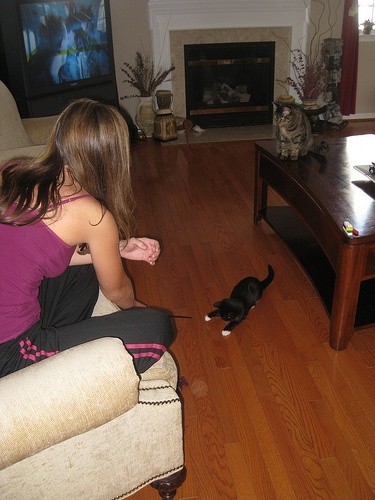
[301,101,323,121]
[135,95,156,137]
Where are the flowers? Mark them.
[119,51,176,101]
[274,0,349,99]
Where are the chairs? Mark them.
[0,81,60,162]
[0,284,188,500]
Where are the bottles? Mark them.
[153,109,178,141]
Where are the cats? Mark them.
[203,263,276,337]
[270,98,330,162]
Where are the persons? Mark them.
[0,99,172,377]
[26,15,67,89]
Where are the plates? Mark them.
[353,165,375,183]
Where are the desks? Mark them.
[254,132,375,350]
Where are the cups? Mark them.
[156,90,171,109]
